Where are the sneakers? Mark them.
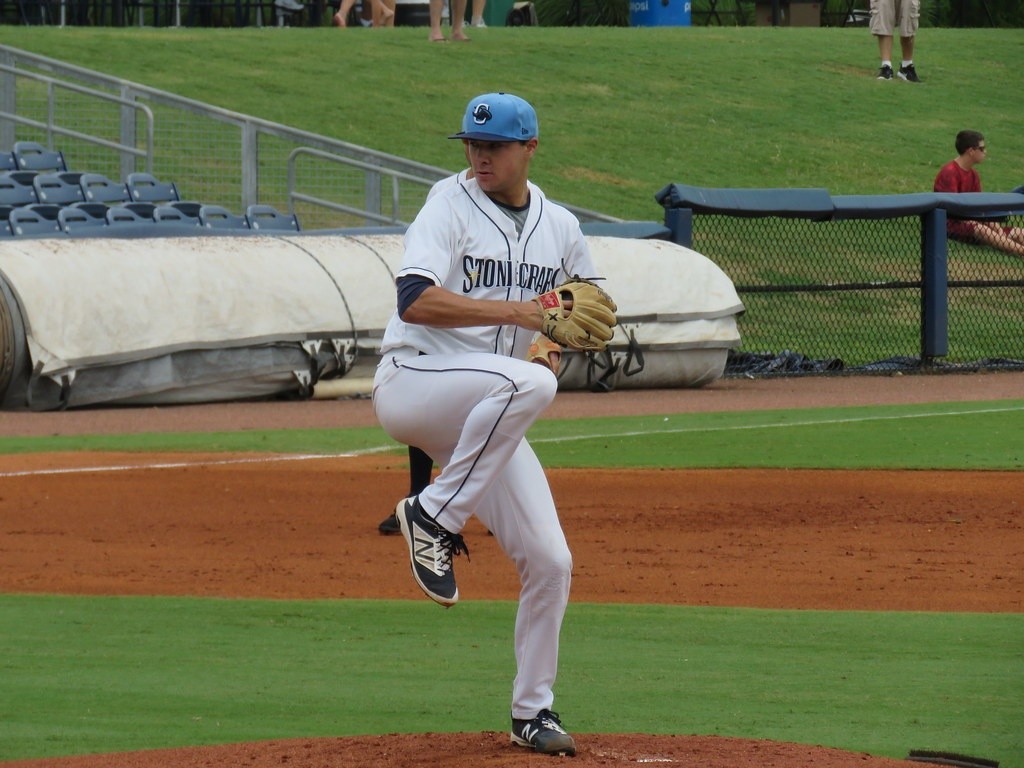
[509,708,576,756]
[876,64,893,80]
[394,494,471,606]
[897,64,920,82]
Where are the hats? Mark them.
[447,92,538,141]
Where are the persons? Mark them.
[333,0,489,44]
[377,114,502,539]
[934,129,1024,258]
[371,90,616,757]
[870,1,921,84]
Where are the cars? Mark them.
[846,9,872,27]
[351,1,444,25]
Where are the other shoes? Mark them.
[378,509,402,532]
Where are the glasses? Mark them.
[972,146,985,152]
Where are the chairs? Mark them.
[0,140,301,236]
